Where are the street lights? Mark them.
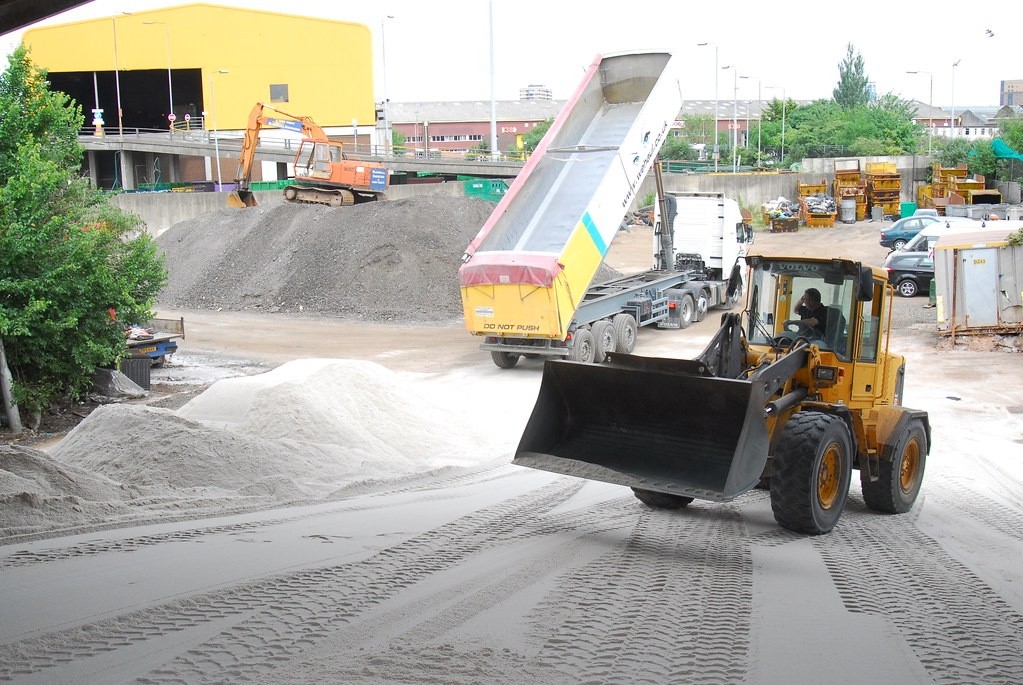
[208,69,230,191]
[907,70,933,157]
[380,13,397,101]
[142,20,175,133]
[722,64,736,172]
[951,58,963,139]
[766,84,786,167]
[113,8,133,137]
[696,42,720,172]
[740,75,760,170]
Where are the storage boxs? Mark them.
[741,159,1003,233]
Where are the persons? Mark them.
[781,288,827,341]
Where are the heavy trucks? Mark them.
[456,46,756,369]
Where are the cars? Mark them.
[880,215,941,251]
[882,252,936,298]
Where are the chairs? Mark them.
[824,307,846,353]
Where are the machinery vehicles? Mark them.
[226,102,389,210]
[512,253,932,536]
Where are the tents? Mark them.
[966,137,1023,182]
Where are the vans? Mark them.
[883,220,1023,272]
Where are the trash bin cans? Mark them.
[929,280,936,306]
[900,202,917,219]
